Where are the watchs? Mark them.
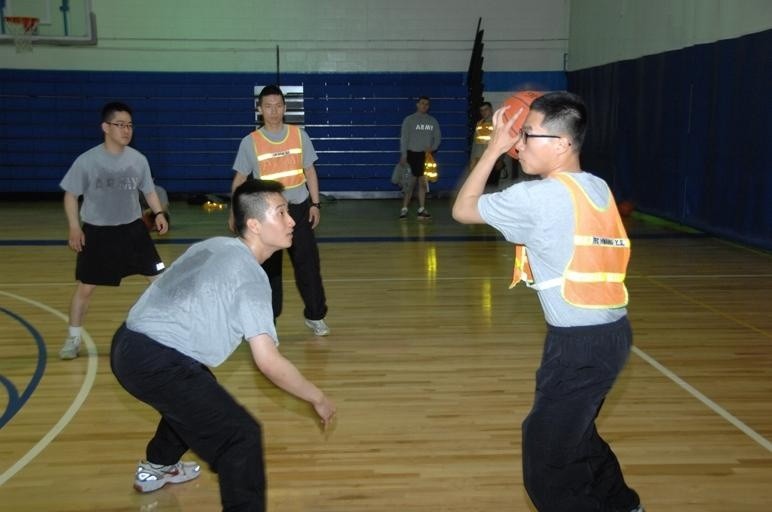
[154,211,165,218]
[311,202,321,209]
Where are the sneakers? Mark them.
[132,458,201,493]
[399,207,408,220]
[416,208,432,219]
[60,331,80,361]
[303,317,331,336]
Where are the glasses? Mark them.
[519,131,571,145]
[102,121,134,129]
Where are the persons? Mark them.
[453,93,645,512]
[401,98,442,220]
[471,102,504,192]
[59,103,169,360]
[112,180,337,512]
[228,86,330,336]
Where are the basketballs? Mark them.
[143,214,152,230]
[501,92,542,159]
[618,202,631,217]
